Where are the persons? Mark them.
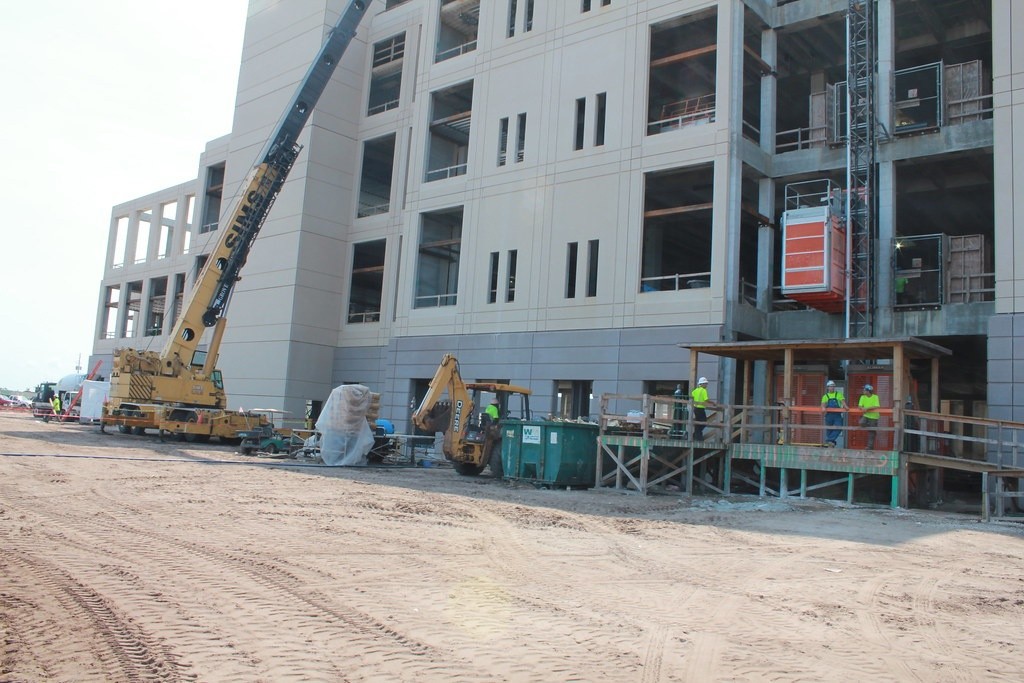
[43,394,60,422]
[486,398,500,422]
[857,385,880,449]
[692,377,718,441]
[821,381,849,449]
[896,266,908,305]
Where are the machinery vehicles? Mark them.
[31,381,58,410]
[411,353,532,482]
[236,409,298,457]
[99,0,374,443]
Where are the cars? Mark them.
[8,395,33,408]
[0,394,13,409]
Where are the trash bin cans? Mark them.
[501,417,657,490]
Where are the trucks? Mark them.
[33,391,82,422]
